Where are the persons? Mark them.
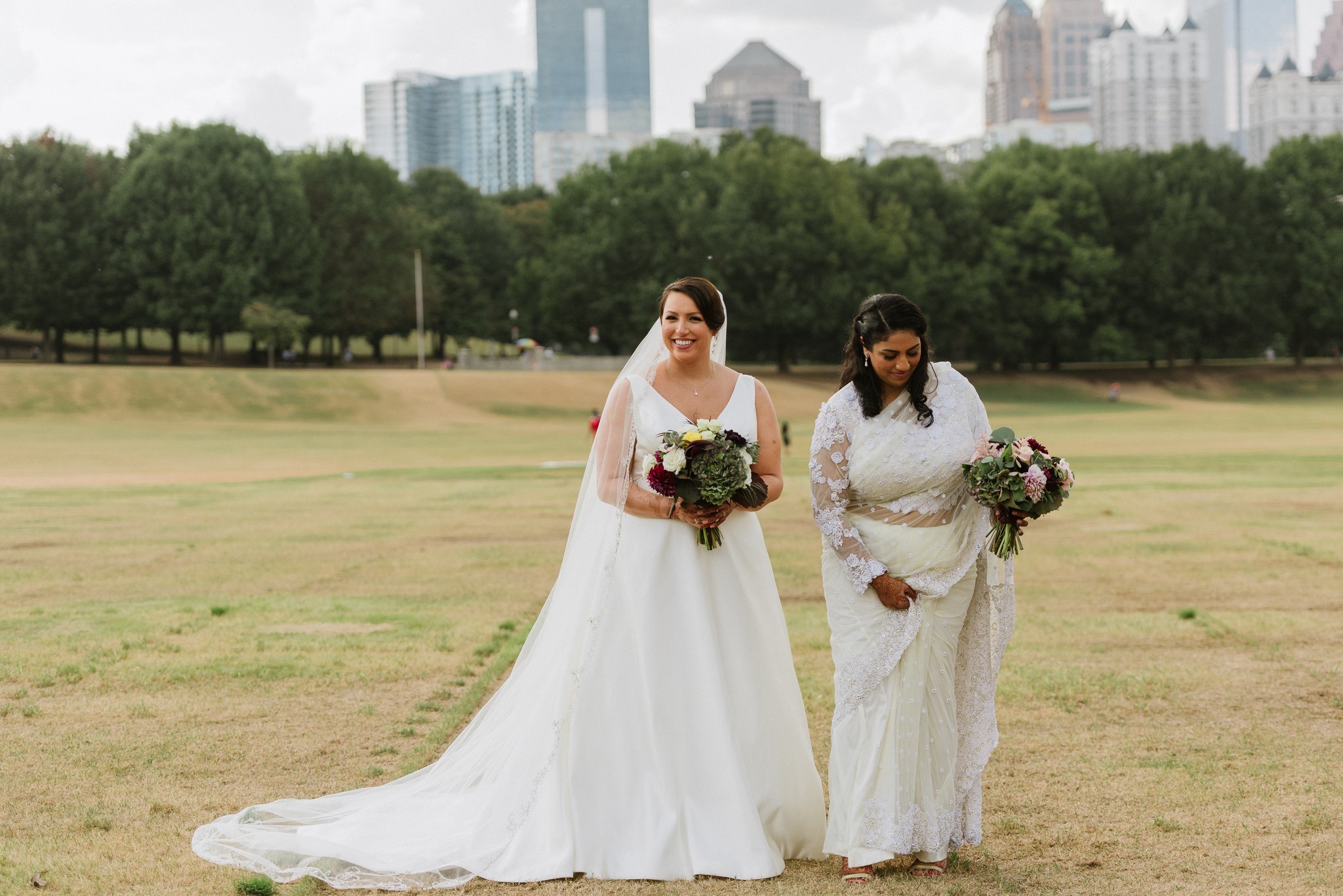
[589,407,601,441]
[810,294,1027,883]
[588,275,827,882]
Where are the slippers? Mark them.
[906,854,949,882]
[839,856,875,887]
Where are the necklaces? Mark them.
[665,358,715,396]
[879,392,908,415]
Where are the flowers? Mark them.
[647,418,758,549]
[962,427,1076,564]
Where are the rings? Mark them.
[694,516,701,524]
[718,510,726,517]
